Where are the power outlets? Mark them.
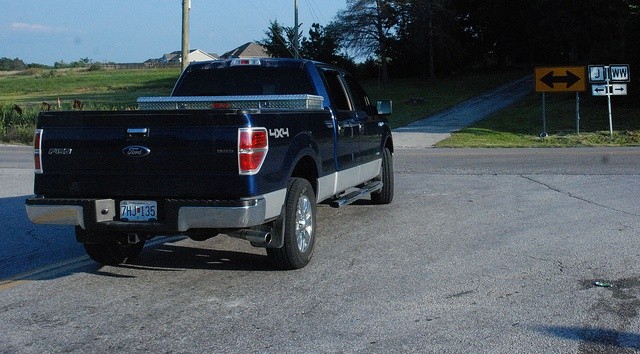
[22,56,395,271]
